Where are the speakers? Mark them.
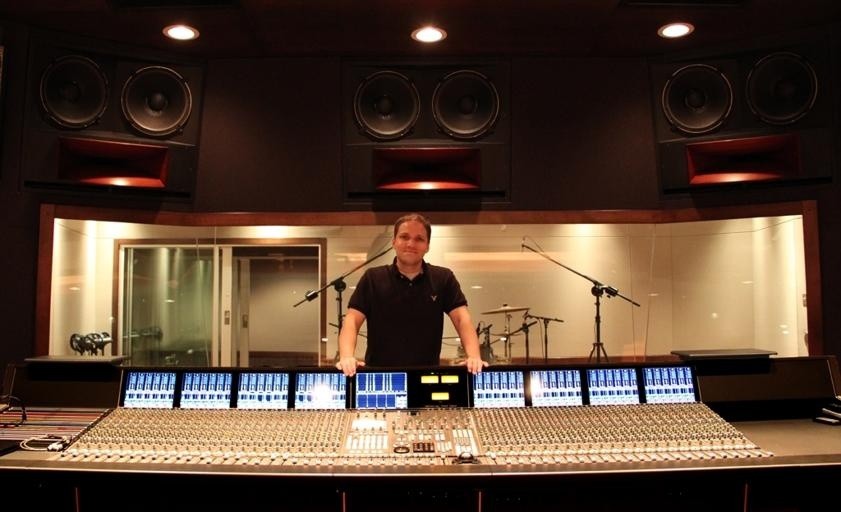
[339,54,514,199]
[646,42,835,195]
[12,35,205,202]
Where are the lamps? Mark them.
[656,15,697,40]
[162,18,199,41]
[410,17,449,45]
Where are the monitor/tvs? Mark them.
[468,365,701,409]
[118,365,408,408]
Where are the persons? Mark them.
[335,212,490,377]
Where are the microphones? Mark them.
[520,238,525,253]
[523,312,528,320]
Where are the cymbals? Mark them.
[480,307,530,314]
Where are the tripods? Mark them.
[525,244,641,364]
